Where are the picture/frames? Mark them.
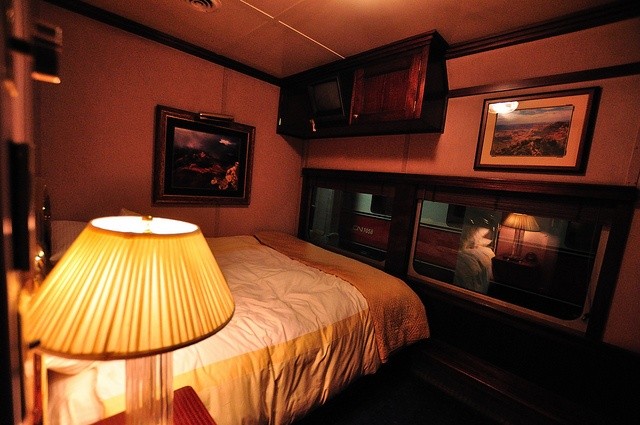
[473,85,603,176]
[150,104,256,207]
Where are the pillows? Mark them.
[459,224,489,251]
[480,237,492,247]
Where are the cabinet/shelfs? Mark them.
[347,28,450,137]
[275,56,348,136]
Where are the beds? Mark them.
[415,241,496,294]
[41,183,434,425]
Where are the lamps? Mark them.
[501,212,541,263]
[26,215,236,425]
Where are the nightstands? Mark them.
[491,254,543,289]
[85,386,215,425]
